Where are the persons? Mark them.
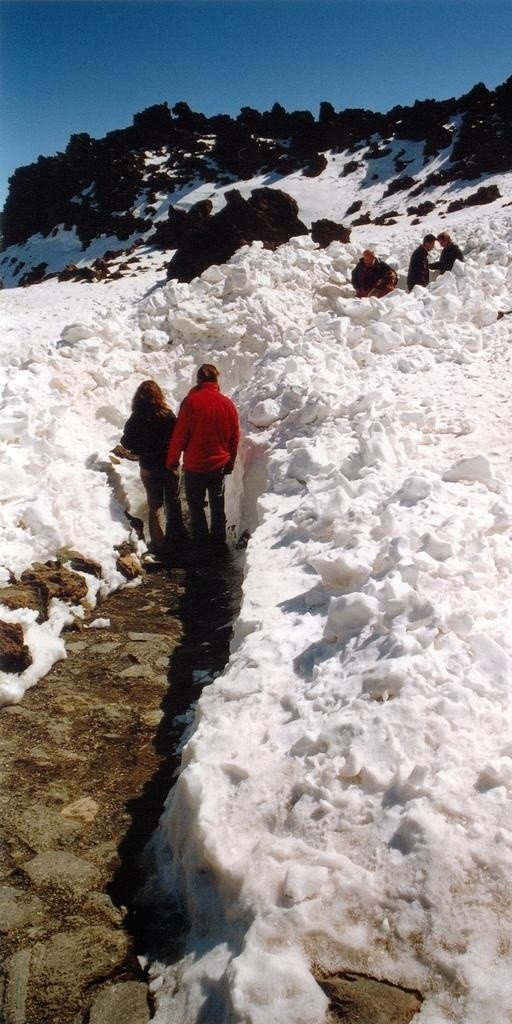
[119,379,191,547]
[350,231,463,299]
[166,363,240,549]
